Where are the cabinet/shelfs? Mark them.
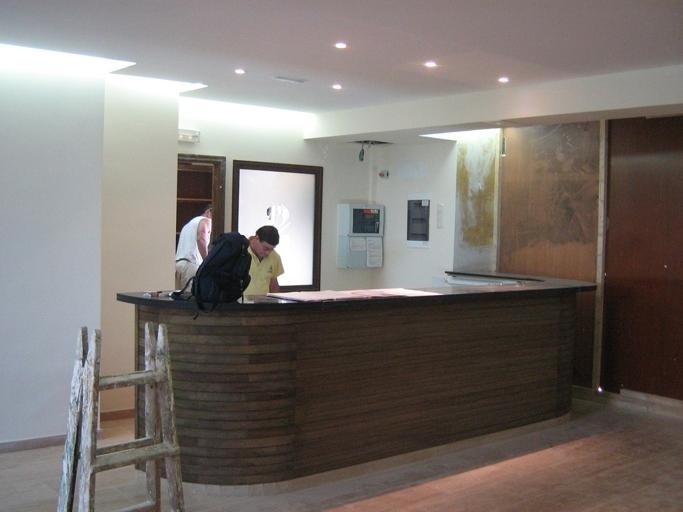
[176,154,226,251]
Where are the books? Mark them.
[266,287,442,303]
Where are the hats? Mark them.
[255,225,280,247]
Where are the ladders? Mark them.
[59,322,184,511]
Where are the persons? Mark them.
[240,225,284,295]
[173,205,211,291]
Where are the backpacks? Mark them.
[190,232,252,304]
